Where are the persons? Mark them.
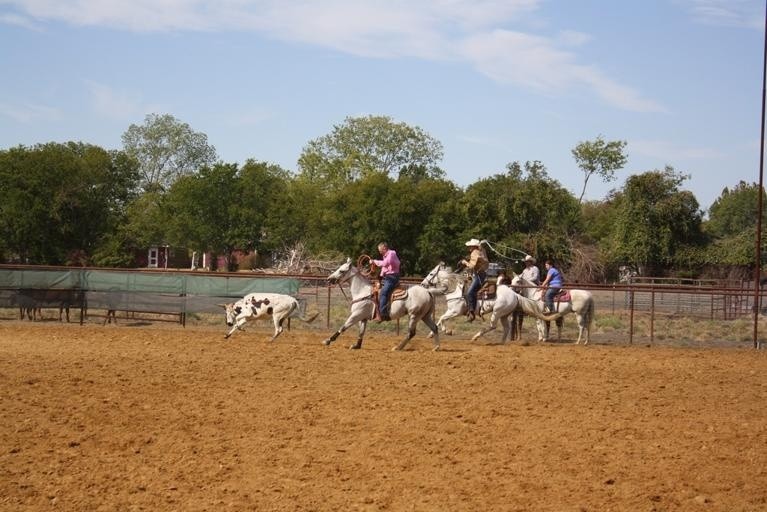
[461,239,489,322]
[369,240,401,323]
[541,259,564,314]
[518,255,541,285]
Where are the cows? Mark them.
[214,292,322,344]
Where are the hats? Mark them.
[465,238,480,246]
[522,255,536,264]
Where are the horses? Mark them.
[421,261,572,347]
[493,270,565,342]
[323,257,451,352]
[510,271,595,348]
